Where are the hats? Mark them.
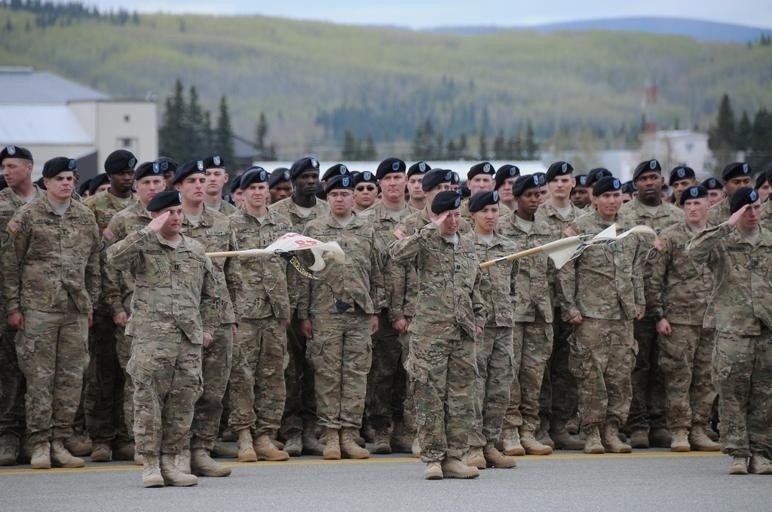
[546,161,573,182]
[634,158,662,181]
[467,162,546,211]
[576,168,635,196]
[223,157,377,199]
[0,145,78,190]
[432,191,462,213]
[376,157,459,191]
[78,150,225,197]
[669,167,723,206]
[722,163,772,214]
[146,191,182,211]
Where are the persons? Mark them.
[0,145,772,470]
[390,192,484,480]
[103,186,214,487]
[687,186,771,475]
[171,158,233,478]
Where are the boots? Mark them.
[729,453,771,474]
[0,428,143,469]
[424,442,516,479]
[238,423,422,461]
[142,429,238,488]
[495,412,720,455]
